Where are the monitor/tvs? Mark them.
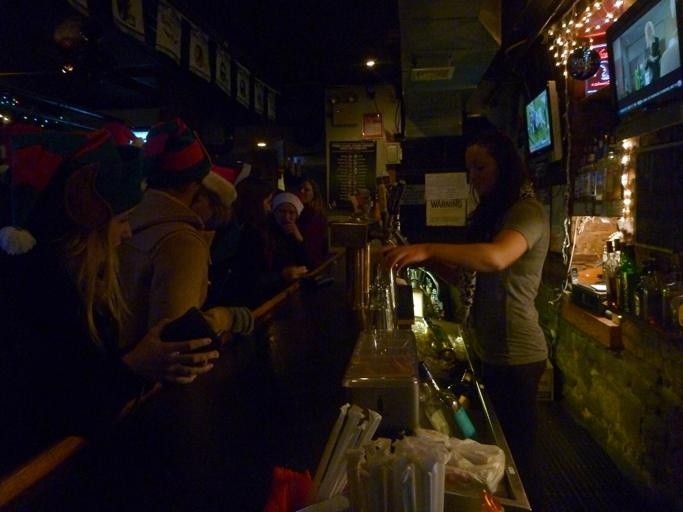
[607,0,683,118]
[525,80,563,169]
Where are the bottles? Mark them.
[413,316,479,441]
[634,38,661,91]
[601,239,683,330]
[579,136,622,202]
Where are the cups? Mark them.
[358,281,393,333]
[367,328,404,364]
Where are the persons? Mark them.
[644,22,662,84]
[375,129,549,512]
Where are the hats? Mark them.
[272,189,305,216]
[1,120,103,257]
[99,122,144,148]
[201,163,252,208]
[145,118,210,187]
